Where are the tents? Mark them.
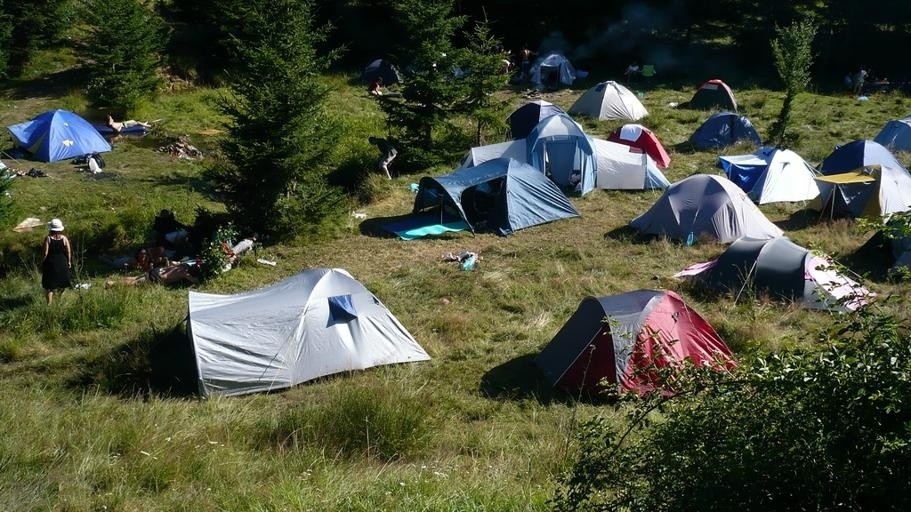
[875,115,911,151]
[535,288,740,399]
[677,236,870,314]
[815,139,911,217]
[358,55,403,83]
[838,213,911,286]
[568,80,649,122]
[5,108,113,163]
[382,101,671,241]
[628,173,785,244]
[688,112,763,151]
[524,49,578,91]
[186,267,432,397]
[688,79,737,110]
[719,139,821,205]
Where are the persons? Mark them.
[107,114,153,132]
[367,75,384,99]
[41,217,72,307]
[369,136,398,180]
[97,204,259,282]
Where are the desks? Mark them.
[814,172,876,221]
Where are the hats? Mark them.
[48,219,64,231]
